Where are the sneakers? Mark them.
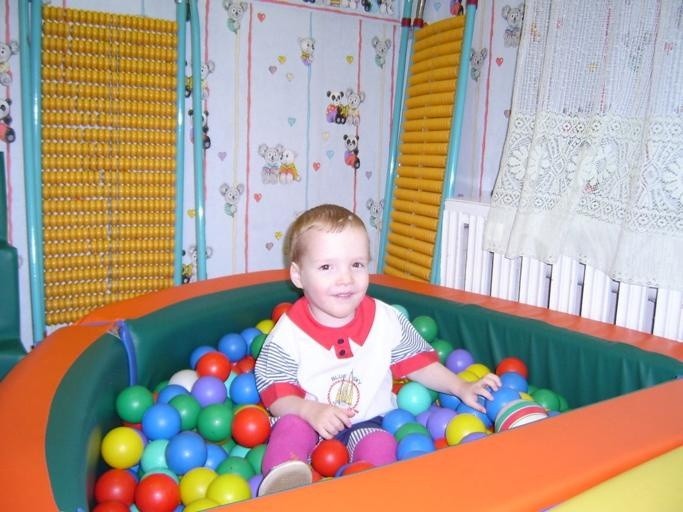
[256,459,313,497]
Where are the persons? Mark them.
[254,203,502,497]
